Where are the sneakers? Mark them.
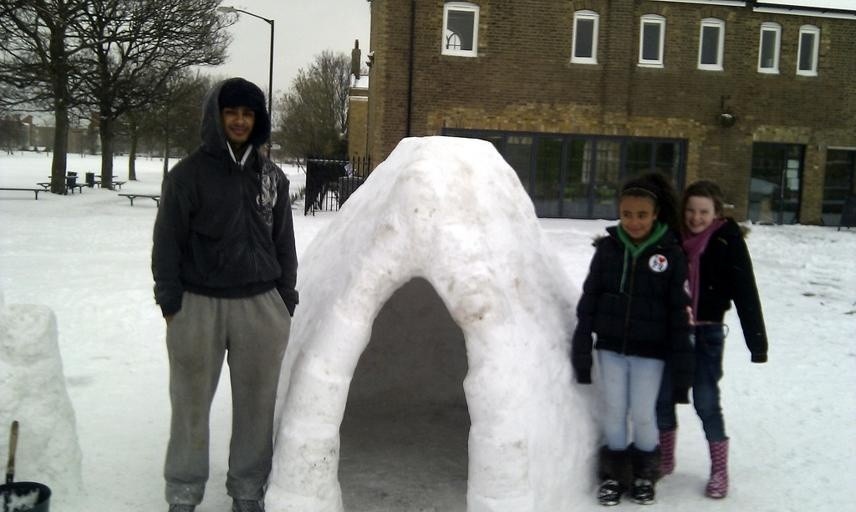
[168,502,195,512]
[232,496,266,511]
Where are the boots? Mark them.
[655,429,676,480]
[596,444,630,506]
[705,436,731,498]
[630,442,657,504]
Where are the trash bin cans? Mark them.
[86,173,94,187]
[67,172,76,189]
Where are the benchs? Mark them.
[36,168,161,208]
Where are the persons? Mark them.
[651,177,768,499]
[151,80,300,512]
[570,174,692,506]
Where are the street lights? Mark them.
[217,6,275,159]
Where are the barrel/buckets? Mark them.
[2,483,51,511]
